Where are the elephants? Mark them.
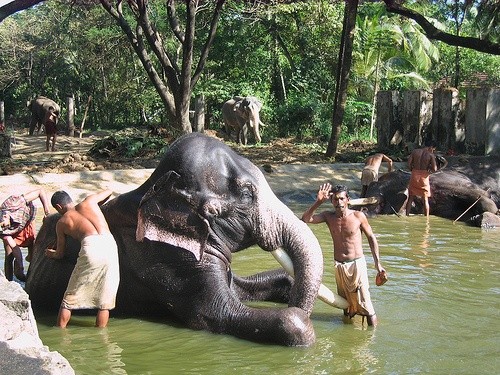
[26,132,354,346]
[347,166,500,227]
[29,96,62,137]
[220,94,269,147]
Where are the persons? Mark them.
[302,183,386,326]
[0,189,51,283]
[406,141,438,217]
[44,106,57,151]
[44,189,121,329]
[359,151,392,198]
